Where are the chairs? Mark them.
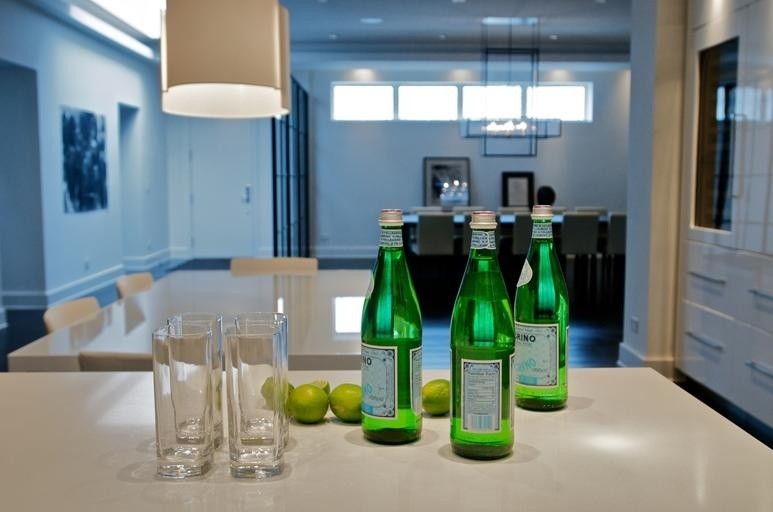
[553,207,566,214]
[555,212,598,255]
[463,212,500,255]
[497,206,530,215]
[513,214,532,254]
[410,206,443,215]
[575,207,608,216]
[43,297,100,334]
[410,213,454,256]
[229,259,318,272]
[287,353,364,371]
[606,214,625,254]
[78,351,153,371]
[452,207,485,213]
[116,271,153,297]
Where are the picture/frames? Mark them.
[423,156,471,206]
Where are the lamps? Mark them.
[159,0,293,120]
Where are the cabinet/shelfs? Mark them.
[674,1,773,428]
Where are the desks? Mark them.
[6,270,375,371]
[401,214,609,247]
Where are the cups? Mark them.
[166,311,223,448]
[222,324,287,479]
[151,325,214,480]
[232,311,288,446]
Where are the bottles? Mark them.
[448,211,516,460]
[514,205,569,411]
[361,209,423,445]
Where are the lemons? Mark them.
[422,379,450,415]
[260,377,295,408]
[310,380,330,394]
[328,384,362,422]
[290,384,329,423]
